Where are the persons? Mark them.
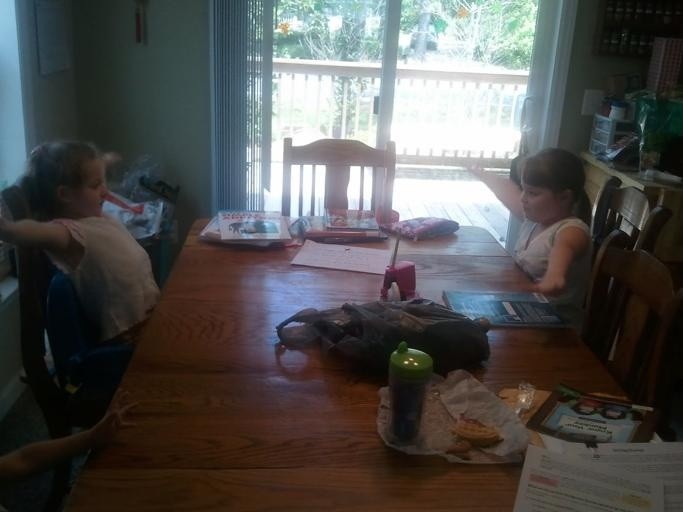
[459,147,595,341]
[0,140,160,428]
[0,387,139,510]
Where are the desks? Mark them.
[48,215,666,512]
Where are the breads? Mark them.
[455,414,500,446]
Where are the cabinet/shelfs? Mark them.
[579,151,682,263]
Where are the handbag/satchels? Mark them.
[274,296,491,381]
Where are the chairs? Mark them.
[280,134,395,215]
[591,173,672,361]
[582,230,682,429]
[0,174,111,438]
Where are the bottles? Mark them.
[386,342,434,447]
[599,0,682,55]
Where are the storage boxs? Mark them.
[588,113,634,156]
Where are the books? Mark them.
[525,381,664,459]
[441,288,572,331]
[197,207,389,250]
